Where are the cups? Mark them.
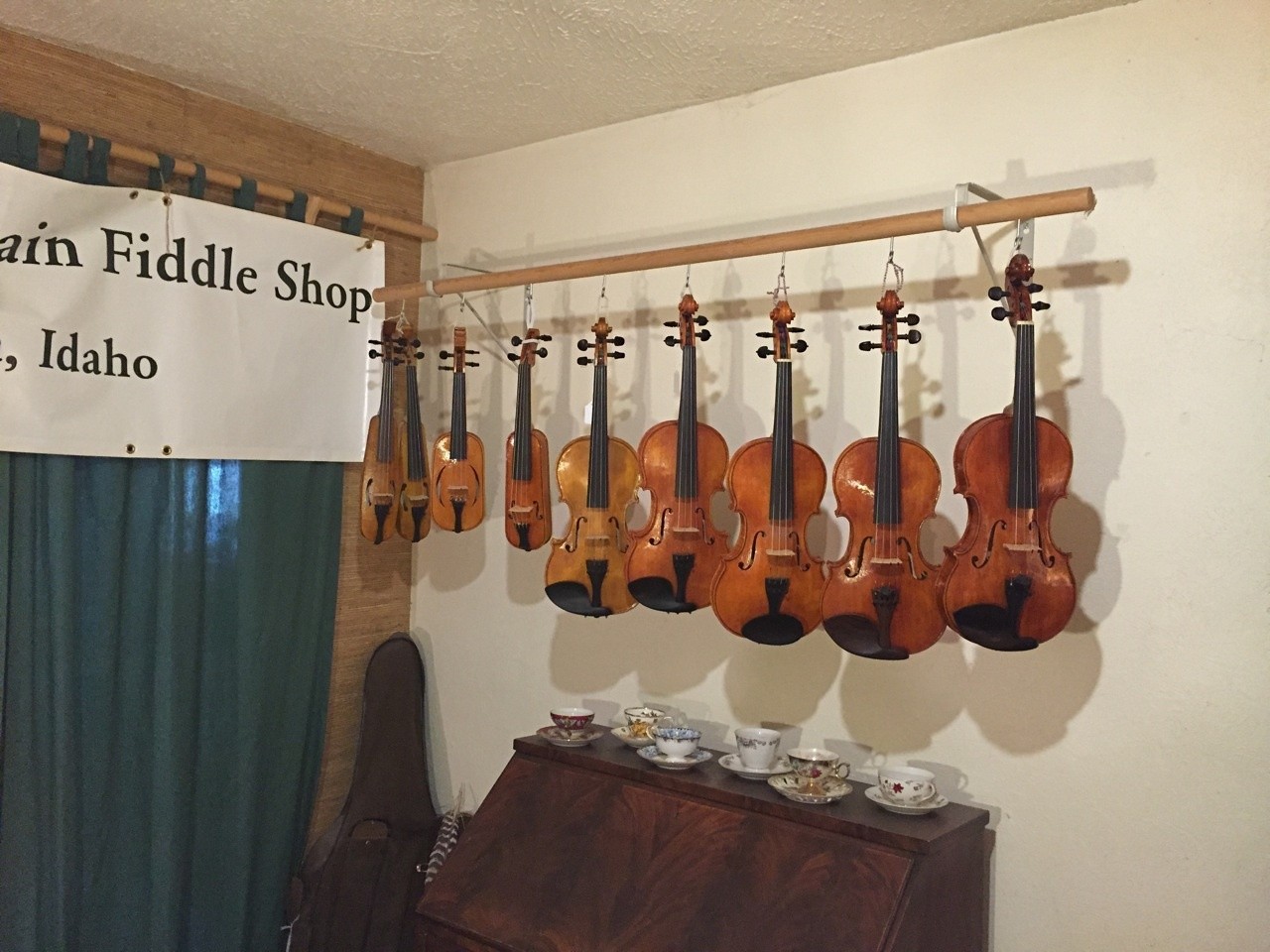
[734,728,781,771]
[549,708,596,739]
[645,726,702,758]
[624,707,675,739]
[786,747,850,793]
[878,766,937,806]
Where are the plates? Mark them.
[767,774,853,804]
[636,746,713,770]
[718,753,793,780]
[864,786,949,815]
[611,726,656,748]
[537,725,603,747]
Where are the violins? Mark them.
[934,253,1076,652]
[819,289,947,661]
[624,294,729,616]
[543,317,642,618]
[505,328,553,552]
[430,326,485,533]
[398,322,431,543]
[359,319,408,545]
[708,301,827,646]
